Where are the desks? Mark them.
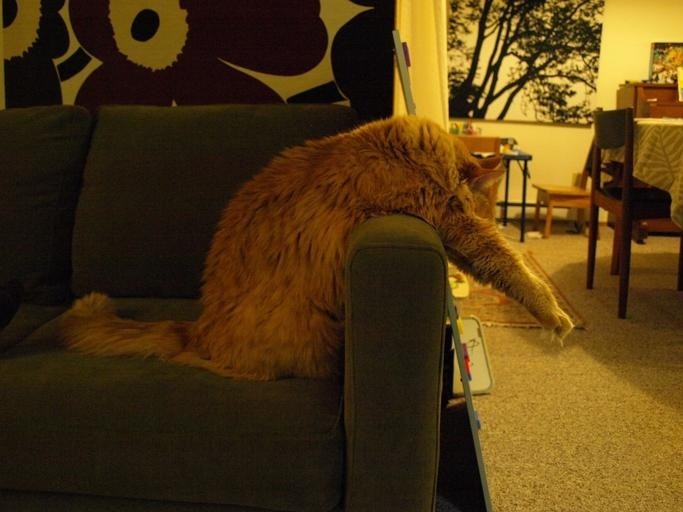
[471,148,532,243]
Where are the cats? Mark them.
[60,114,576,383]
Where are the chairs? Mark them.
[458,136,500,157]
[532,132,595,238]
[586,107,683,318]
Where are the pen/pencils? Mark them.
[457,314,473,380]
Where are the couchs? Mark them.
[3,105,448,512]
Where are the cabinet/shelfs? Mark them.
[617,83,678,117]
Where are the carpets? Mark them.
[449,249,586,330]
